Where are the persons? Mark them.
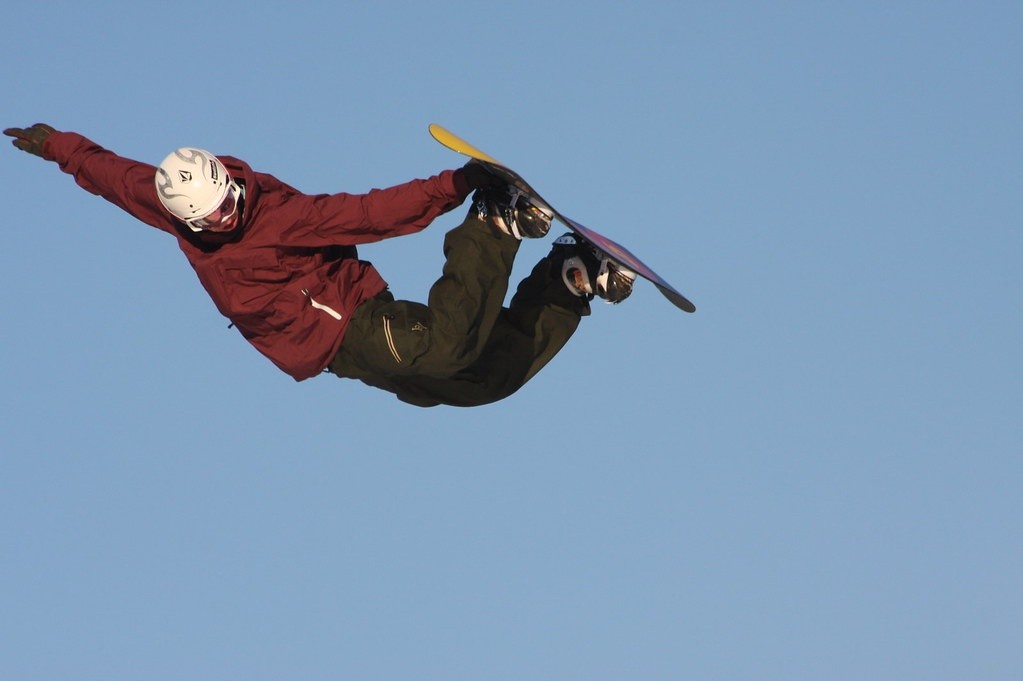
[2,123,639,408]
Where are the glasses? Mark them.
[196,186,241,232]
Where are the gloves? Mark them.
[459,160,505,195]
[3,120,56,158]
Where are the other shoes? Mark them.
[469,185,551,240]
[554,230,634,304]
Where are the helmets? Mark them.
[154,149,238,233]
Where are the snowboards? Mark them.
[427,123,696,315]
